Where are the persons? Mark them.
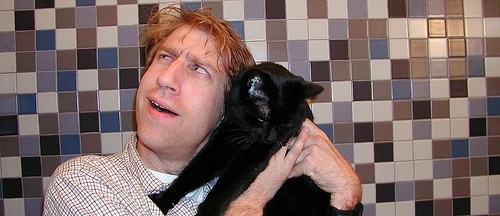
[42,2,364,216]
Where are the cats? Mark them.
[147,61,337,216]
